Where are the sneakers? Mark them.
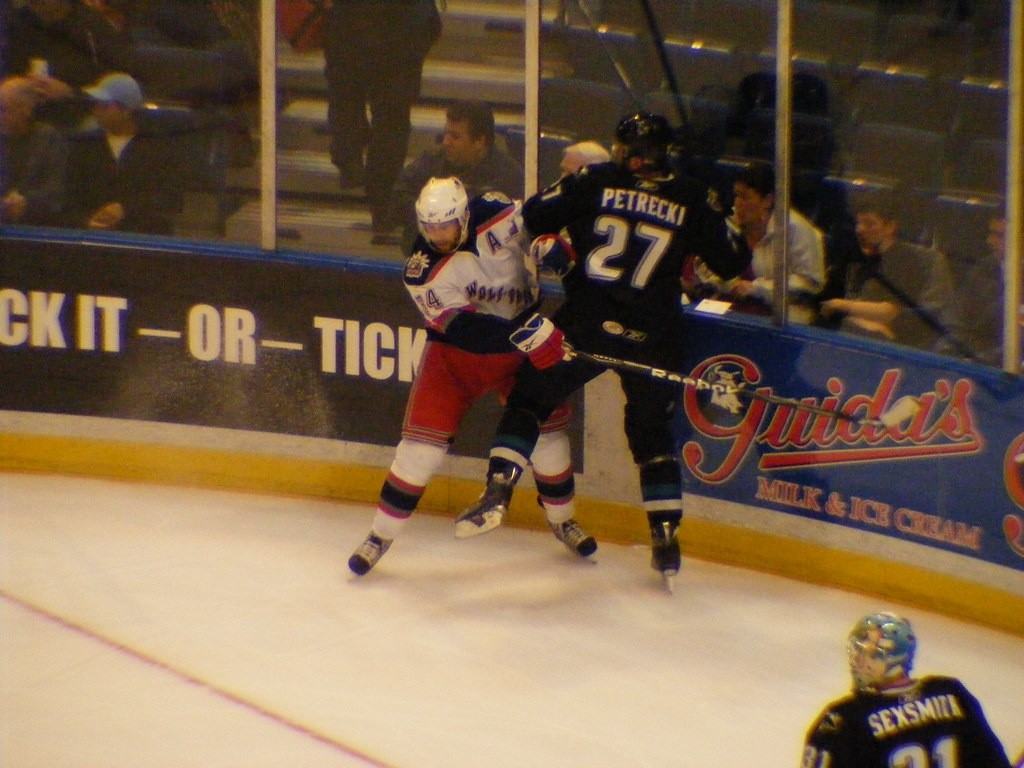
[346,529,395,582]
[651,519,681,595]
[454,466,520,538]
[537,495,598,565]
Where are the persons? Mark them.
[451,109,755,595]
[0,1,1024,375]
[799,610,1014,768]
[341,140,615,585]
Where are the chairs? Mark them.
[480,0,1005,365]
[0,0,260,240]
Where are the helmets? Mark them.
[846,611,916,679]
[614,113,676,161]
[414,177,470,243]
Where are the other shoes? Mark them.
[340,172,365,188]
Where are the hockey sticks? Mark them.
[639,0,690,127]
[865,254,1024,406]
[568,349,923,428]
[576,0,647,115]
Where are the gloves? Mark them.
[509,313,573,369]
[729,234,756,281]
[528,234,577,277]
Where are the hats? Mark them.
[79,73,144,112]
